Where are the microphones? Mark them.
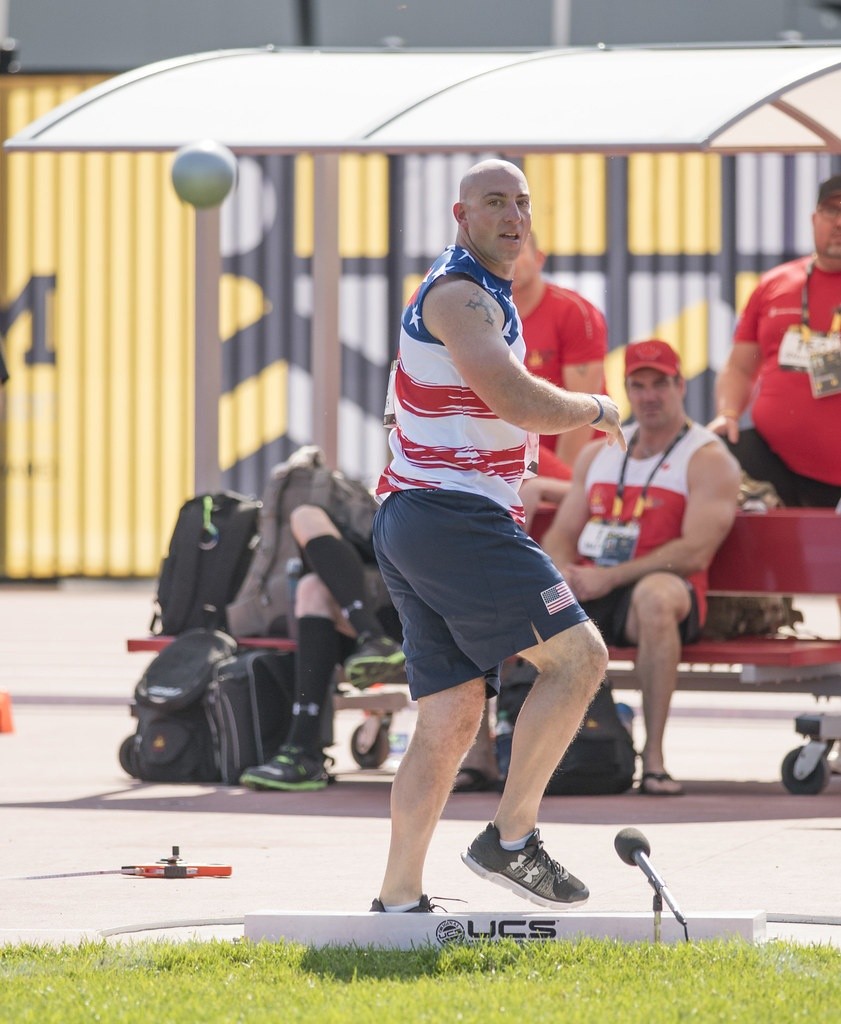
[614,827,688,927]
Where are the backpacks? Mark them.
[494,656,635,794]
[120,445,378,785]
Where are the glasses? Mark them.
[817,202,841,219]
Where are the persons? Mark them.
[704,175,841,509]
[540,339,741,793]
[239,231,606,791]
[370,159,629,913]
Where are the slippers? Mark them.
[639,772,683,794]
[452,767,502,791]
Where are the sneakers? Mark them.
[240,740,331,791]
[461,822,589,909]
[370,890,467,913]
[348,638,406,689]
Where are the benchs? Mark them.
[128,501,841,793]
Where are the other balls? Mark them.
[171,142,236,207]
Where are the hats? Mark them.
[625,341,682,377]
[817,176,841,200]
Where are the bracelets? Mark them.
[589,395,604,424]
